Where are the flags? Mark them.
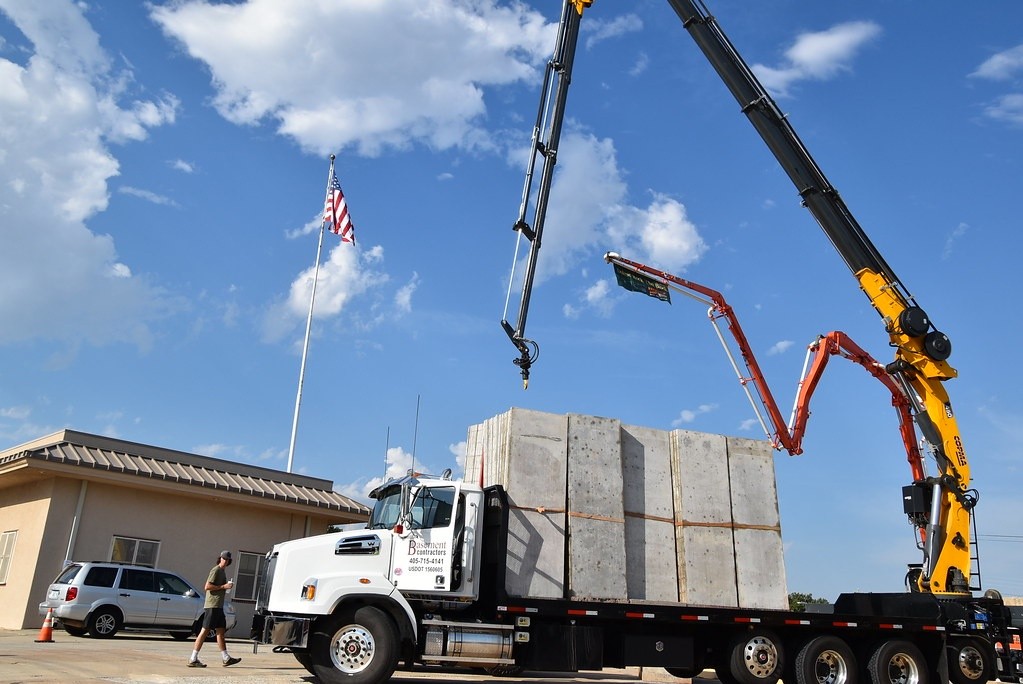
[321,166,357,246]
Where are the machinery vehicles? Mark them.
[494,0,1023,683]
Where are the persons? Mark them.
[187,550,242,668]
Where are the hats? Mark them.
[220,550,232,559]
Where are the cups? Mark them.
[225,581,234,594]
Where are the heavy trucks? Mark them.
[251,466,953,684]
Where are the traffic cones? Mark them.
[33,606,56,643]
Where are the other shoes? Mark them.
[222,657,241,667]
[186,659,207,668]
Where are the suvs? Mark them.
[38,561,238,639]
